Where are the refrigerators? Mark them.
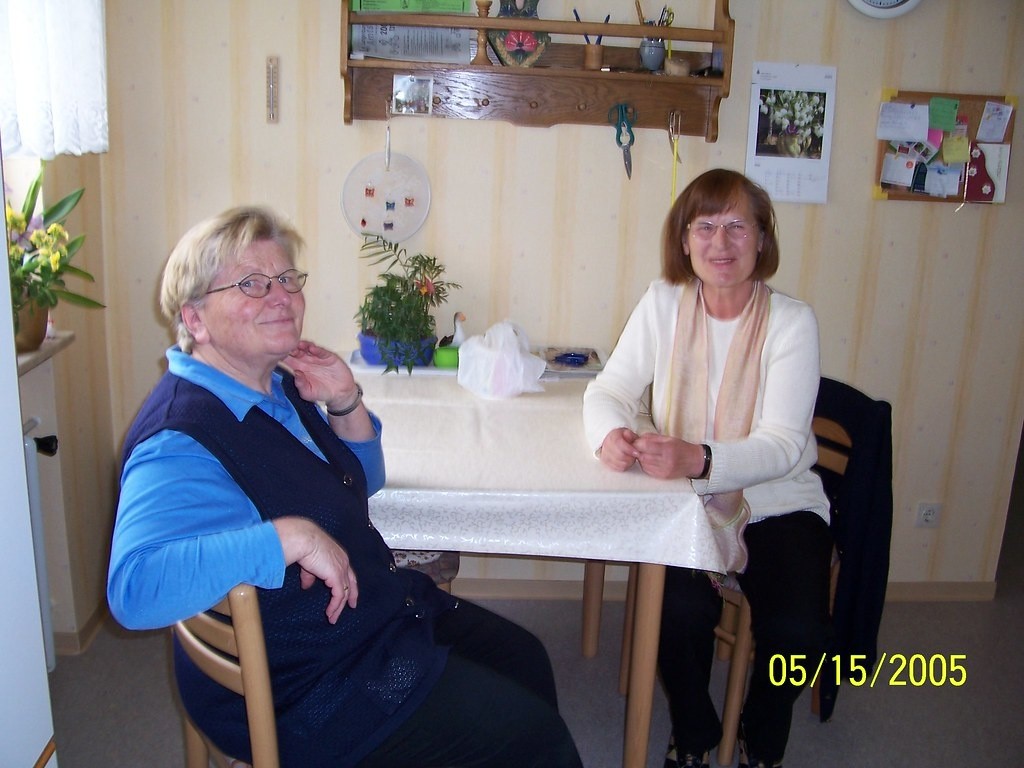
[0,137,57,768]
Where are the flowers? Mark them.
[7,164,107,337]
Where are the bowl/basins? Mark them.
[433,346,458,368]
[665,58,690,76]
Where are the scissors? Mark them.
[654,10,674,41]
[608,102,638,179]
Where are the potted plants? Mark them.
[359,232,464,377]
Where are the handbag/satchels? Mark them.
[456,323,546,399]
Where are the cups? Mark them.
[585,44,603,70]
[639,41,665,71]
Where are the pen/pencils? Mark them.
[595,15,611,44]
[636,1,671,42]
[573,8,590,43]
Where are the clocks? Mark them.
[848,0,923,18]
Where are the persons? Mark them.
[583,168,832,768]
[106,199,583,768]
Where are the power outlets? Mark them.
[914,502,942,527]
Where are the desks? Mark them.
[346,360,728,768]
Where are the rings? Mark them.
[344,586,348,590]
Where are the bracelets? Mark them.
[324,383,363,416]
[688,444,712,479]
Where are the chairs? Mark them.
[618,372,891,768]
[171,584,280,768]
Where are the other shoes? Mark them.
[663,732,710,768]
[737,710,783,768]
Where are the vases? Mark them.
[13,296,50,350]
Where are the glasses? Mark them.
[204,269,309,299]
[687,219,761,239]
